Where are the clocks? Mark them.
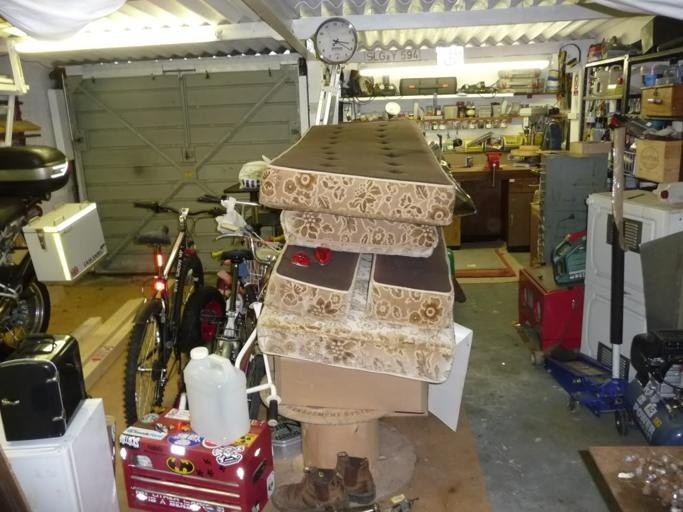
[313,17,357,64]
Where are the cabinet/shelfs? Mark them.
[578,54,627,143]
[505,171,540,248]
[626,48,683,140]
[334,64,568,154]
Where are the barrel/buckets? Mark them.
[186,347,251,448]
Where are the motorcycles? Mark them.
[1,145,72,357]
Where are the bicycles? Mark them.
[197,198,265,359]
[124,202,226,428]
[225,183,283,417]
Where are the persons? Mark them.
[588,104,610,140]
[540,107,562,151]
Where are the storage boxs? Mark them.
[21,200,108,282]
[0,334,86,441]
[119,407,276,512]
[639,16,682,50]
[638,84,680,119]
[633,140,681,183]
